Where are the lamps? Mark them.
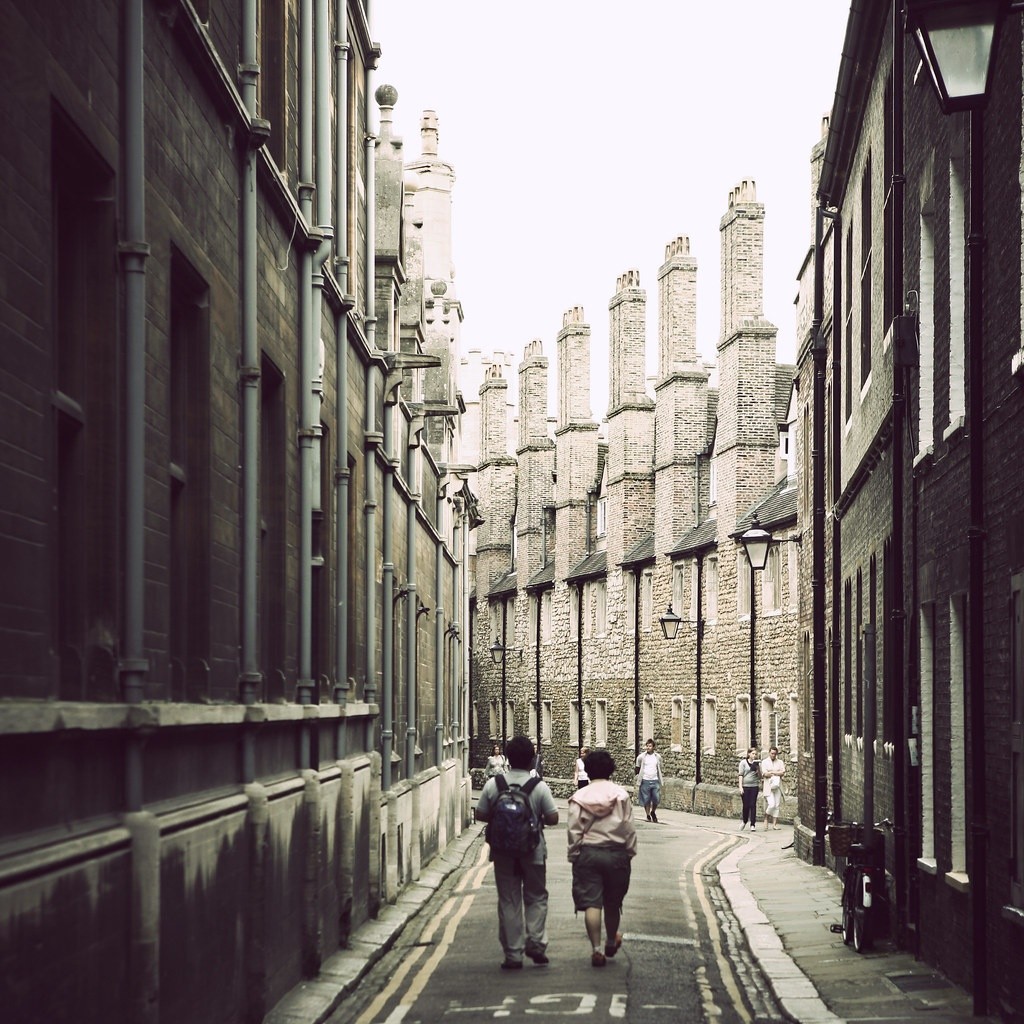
[490,637,523,664]
[742,512,802,571]
[659,603,705,640]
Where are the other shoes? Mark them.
[764,821,768,831]
[773,826,781,830]
[739,820,746,831]
[501,957,523,969]
[526,951,548,964]
[750,823,755,831]
[647,814,651,820]
[605,932,623,958]
[651,811,658,823]
[593,953,606,967]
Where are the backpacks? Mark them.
[489,774,543,854]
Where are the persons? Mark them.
[484,745,508,778]
[567,750,638,965]
[475,736,559,967]
[635,739,664,822]
[762,747,785,830]
[574,747,589,789]
[739,748,760,831]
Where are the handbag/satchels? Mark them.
[530,769,541,779]
[769,774,780,790]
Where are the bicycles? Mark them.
[825,812,893,953]
